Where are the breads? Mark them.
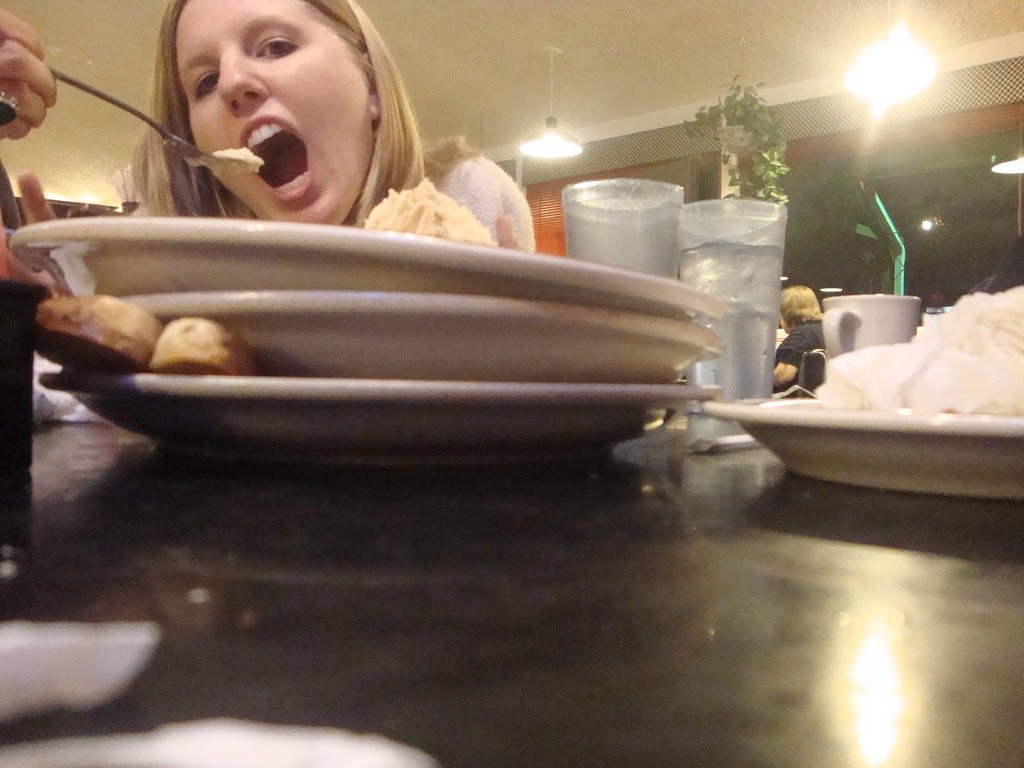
[33,292,161,374]
[145,318,258,376]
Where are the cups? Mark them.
[822,294,922,359]
[560,178,684,279]
[681,197,789,456]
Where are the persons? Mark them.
[0,1,537,296]
[772,286,826,398]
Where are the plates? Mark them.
[6,216,729,469]
[703,400,1024,500]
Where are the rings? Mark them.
[0,91,18,127]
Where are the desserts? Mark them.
[361,176,494,244]
[211,147,265,169]
